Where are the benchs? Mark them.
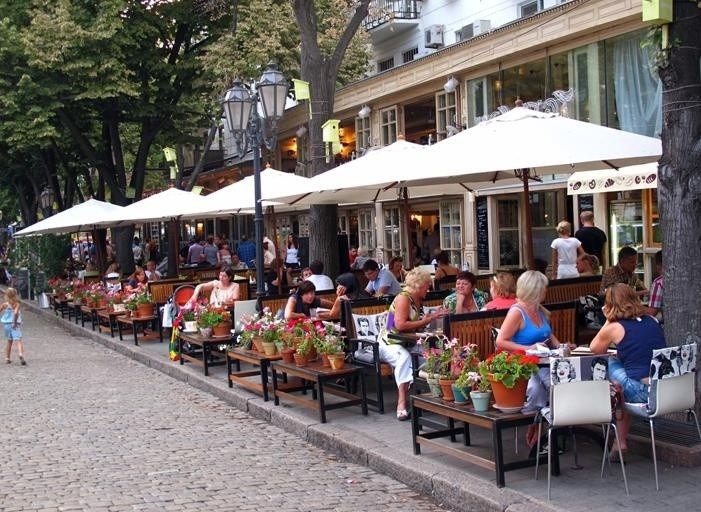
[349,270,643,440]
[69,268,348,327]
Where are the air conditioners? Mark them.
[460,19,491,43]
[423,23,446,49]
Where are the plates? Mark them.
[182,329,198,333]
[492,400,529,415]
[572,346,597,355]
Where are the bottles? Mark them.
[559,342,570,357]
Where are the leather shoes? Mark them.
[396,410,408,420]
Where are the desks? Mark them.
[177,330,242,377]
[48,291,161,346]
[228,346,306,401]
[408,392,561,488]
[270,357,369,424]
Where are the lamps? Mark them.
[359,103,372,119]
[444,74,461,93]
[296,124,308,138]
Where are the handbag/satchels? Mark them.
[355,349,374,364]
[579,295,602,330]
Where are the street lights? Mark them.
[38,184,56,218]
[218,56,291,315]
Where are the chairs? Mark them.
[530,350,631,501]
[601,340,701,489]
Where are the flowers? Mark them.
[179,300,231,329]
[48,276,148,309]
[232,307,347,355]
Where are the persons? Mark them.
[180,235,257,270]
[72,237,163,293]
[250,233,407,321]
[379,212,665,463]
[677,345,693,375]
[191,267,240,308]
[0,287,26,365]
